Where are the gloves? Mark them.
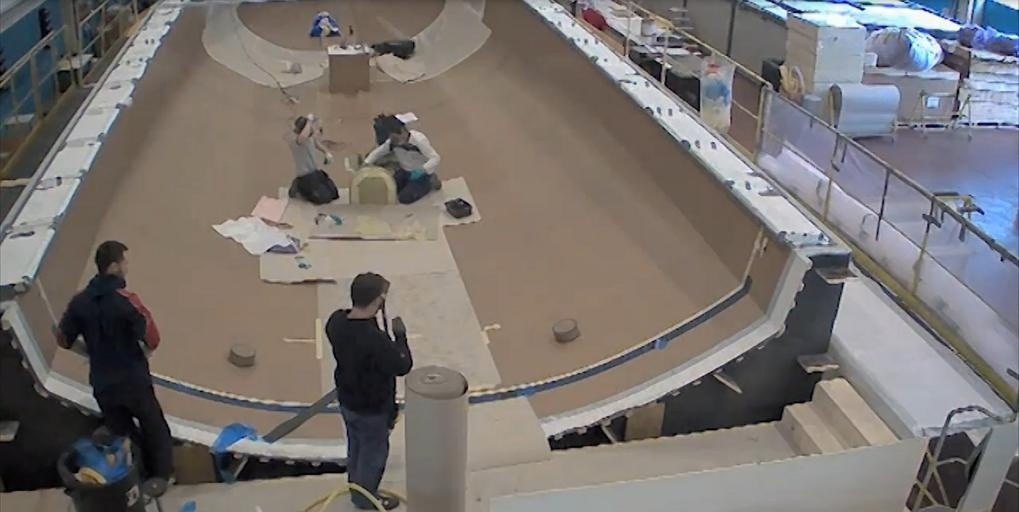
[409,168,427,183]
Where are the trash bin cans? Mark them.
[758,55,785,98]
[57,433,146,512]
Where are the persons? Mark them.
[324,271,413,508]
[289,114,339,205]
[361,120,442,203]
[54,240,178,485]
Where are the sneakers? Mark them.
[429,171,442,191]
[349,493,399,511]
[288,176,301,199]
